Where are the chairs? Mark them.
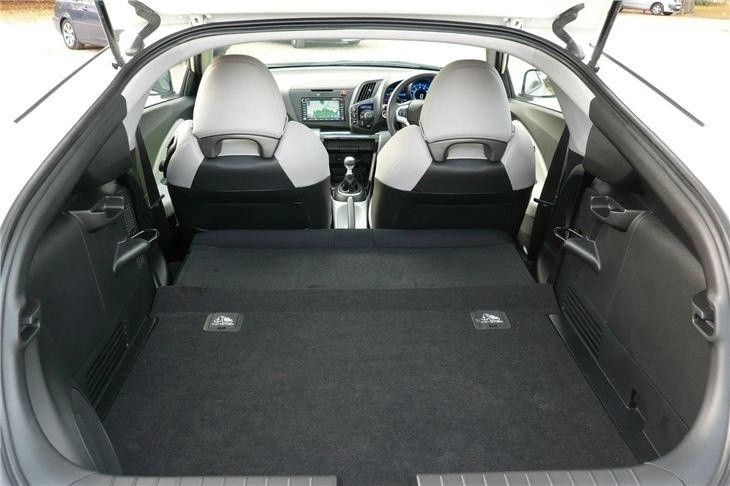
[162,53,333,227]
[370,59,537,233]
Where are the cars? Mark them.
[47,0,109,52]
[284,38,365,50]
[0,1,730,485]
[620,0,685,15]
